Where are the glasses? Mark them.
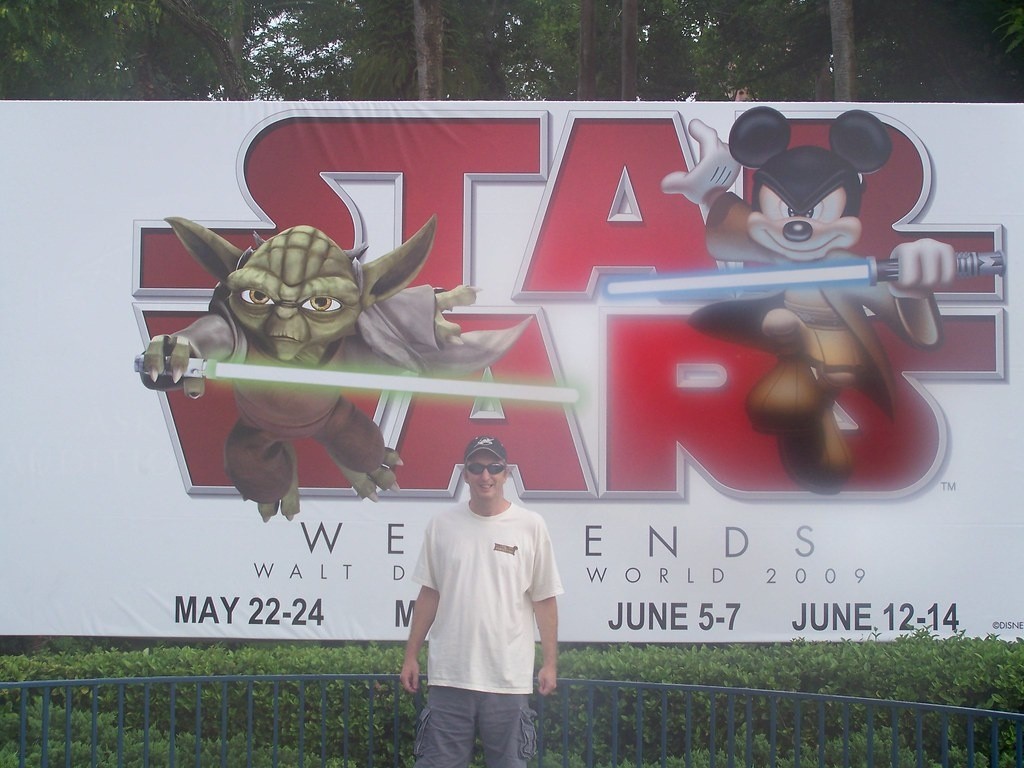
[467,462,505,475]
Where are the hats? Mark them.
[464,435,506,463]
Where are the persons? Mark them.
[400,435,564,768]
